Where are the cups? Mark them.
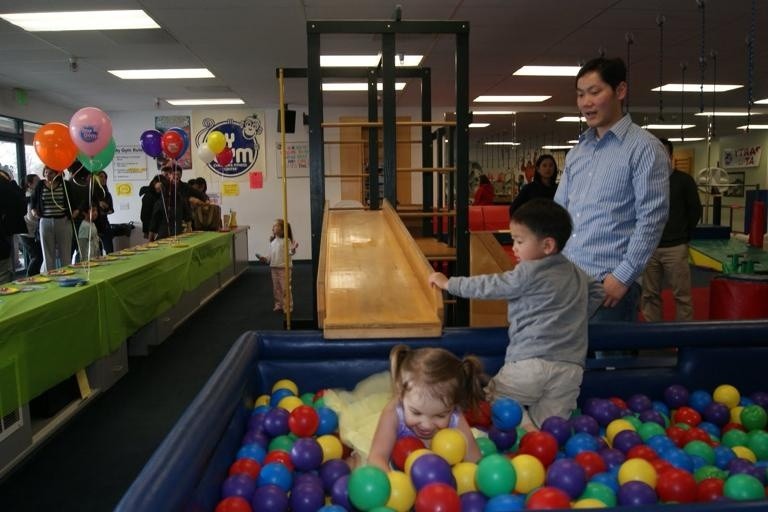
[224,215,229,230]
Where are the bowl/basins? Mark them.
[55,278,82,287]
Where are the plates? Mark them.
[67,262,101,268]
[90,256,118,261]
[48,270,76,275]
[0,287,21,295]
[109,231,202,255]
[15,277,53,285]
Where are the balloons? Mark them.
[140,127,190,161]
[200,130,233,167]
[32,107,116,177]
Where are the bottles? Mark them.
[230,211,237,227]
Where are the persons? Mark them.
[255,219,299,315]
[365,341,495,475]
[140,164,208,243]
[472,154,559,219]
[429,193,607,436]
[633,139,702,323]
[0,160,115,286]
[549,59,669,357]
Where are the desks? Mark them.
[0,220,252,478]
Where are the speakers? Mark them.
[278,110,296,133]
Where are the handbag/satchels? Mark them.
[193,203,221,231]
[39,197,45,218]
